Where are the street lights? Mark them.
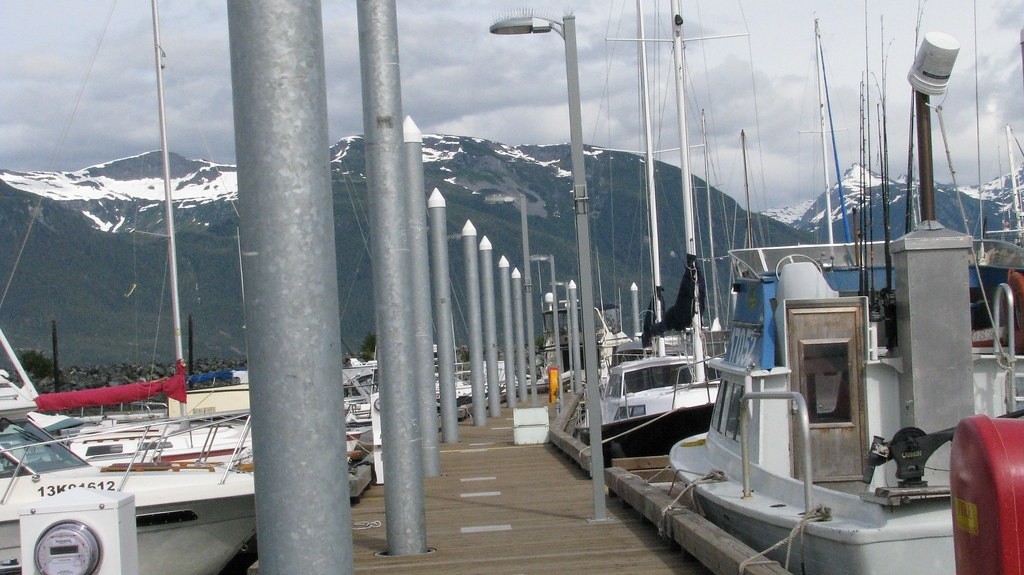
[529,254,564,401]
[481,193,540,408]
[489,15,617,523]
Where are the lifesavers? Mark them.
[1002,267,1024,354]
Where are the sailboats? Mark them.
[568,0,1024,575]
[1,0,549,575]
